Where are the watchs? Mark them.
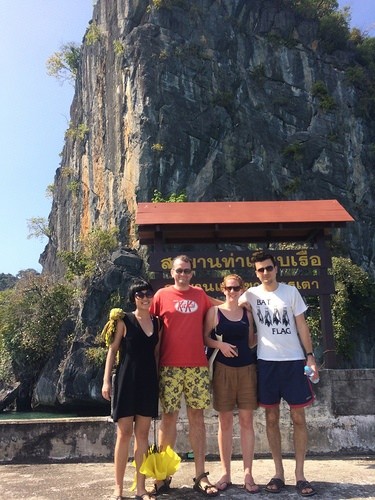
[306,352,316,357]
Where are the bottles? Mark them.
[305,366,320,383]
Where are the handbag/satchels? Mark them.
[206,305,223,380]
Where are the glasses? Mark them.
[256,264,275,273]
[225,286,241,292]
[134,289,154,300]
[172,268,192,275]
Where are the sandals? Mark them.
[158,476,172,492]
[193,472,219,497]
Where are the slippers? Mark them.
[296,481,315,496]
[111,494,122,500]
[216,480,232,490]
[243,482,260,494]
[135,491,156,500]
[265,478,285,492]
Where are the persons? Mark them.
[203,274,259,493]
[132,254,252,496]
[101,281,163,500]
[237,254,319,496]
